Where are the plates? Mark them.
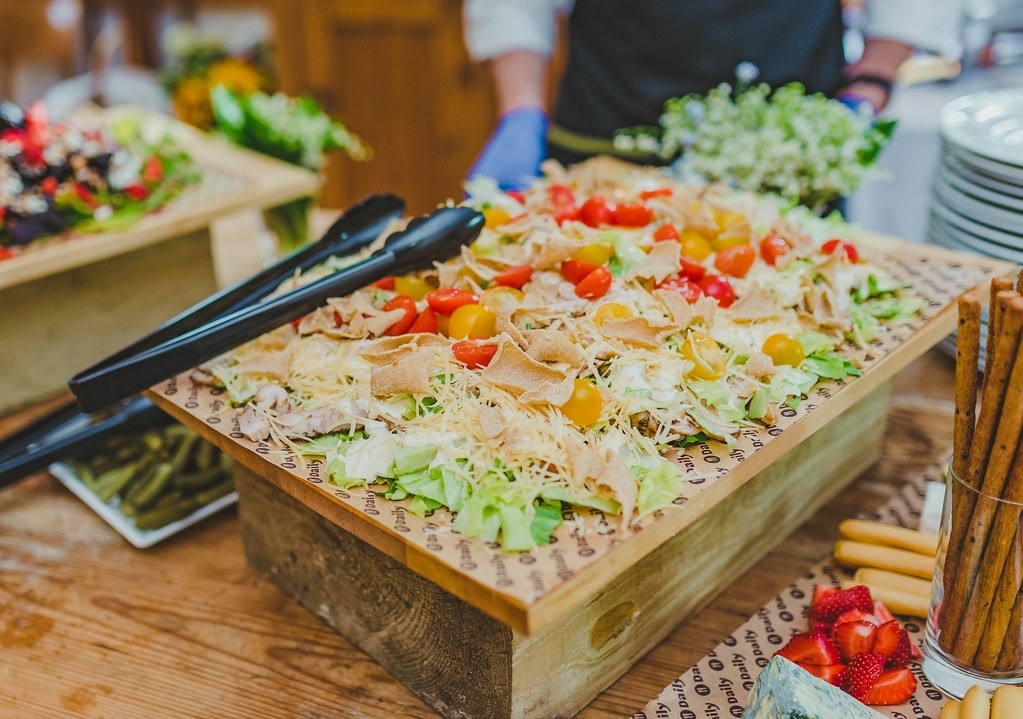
[920,87,1023,374]
[47,458,239,549]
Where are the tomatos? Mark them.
[293,187,857,423]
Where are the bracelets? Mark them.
[848,72,891,107]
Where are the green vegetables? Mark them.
[219,256,929,550]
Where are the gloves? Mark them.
[839,97,874,127]
[465,110,549,200]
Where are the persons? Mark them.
[461,0,952,199]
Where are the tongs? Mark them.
[67,193,485,414]
[1,401,172,489]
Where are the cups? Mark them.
[922,452,1022,704]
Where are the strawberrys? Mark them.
[772,587,917,704]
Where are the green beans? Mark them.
[76,421,233,529]
